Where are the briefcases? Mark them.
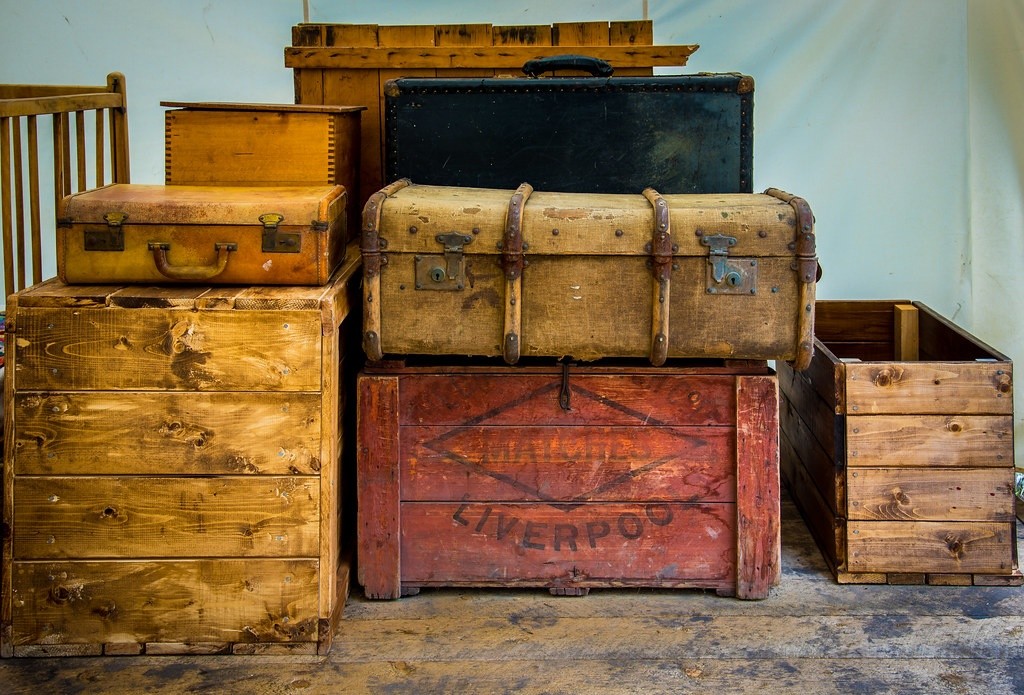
[55,185,348,285]
[384,55,755,199]
